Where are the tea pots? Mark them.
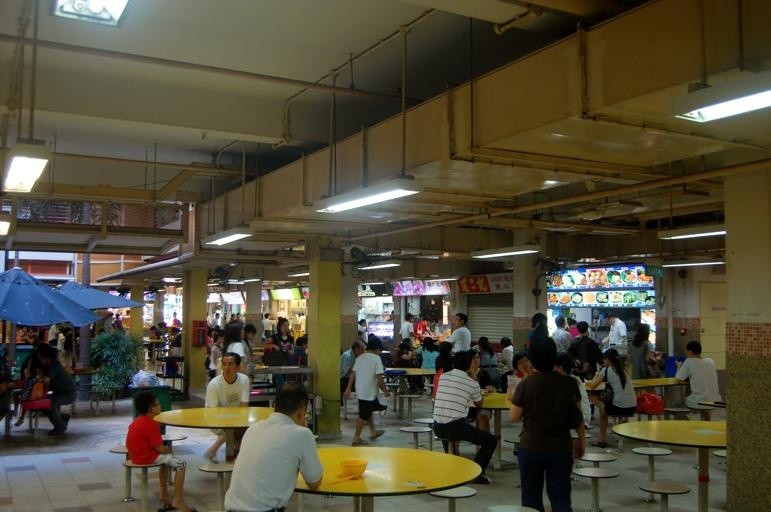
[507,374,522,403]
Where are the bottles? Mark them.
[429,318,441,337]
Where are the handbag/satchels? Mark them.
[565,405,582,429]
[636,392,664,414]
[600,383,613,401]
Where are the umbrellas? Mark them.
[54,280,145,367]
[0,266,104,362]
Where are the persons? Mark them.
[224,382,324,512]
[122,310,131,328]
[0,322,79,436]
[675,339,722,422]
[341,313,515,486]
[149,312,309,383]
[205,352,252,463]
[126,392,199,512]
[113,314,122,329]
[509,337,584,511]
[526,312,652,448]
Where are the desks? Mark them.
[144,337,307,382]
[153,407,314,463]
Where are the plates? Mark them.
[546,270,656,306]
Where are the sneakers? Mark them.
[368,430,383,439]
[592,439,607,447]
[351,439,367,446]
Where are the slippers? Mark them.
[158,503,173,512]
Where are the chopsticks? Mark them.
[331,473,366,484]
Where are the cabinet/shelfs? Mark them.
[248,365,317,434]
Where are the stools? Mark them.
[572,468,619,512]
[0,367,117,441]
[161,433,187,485]
[506,437,523,487]
[109,448,134,502]
[487,505,539,512]
[199,464,234,511]
[428,485,477,511]
[631,447,672,503]
[400,372,726,447]
[122,460,162,512]
[344,348,436,418]
[639,480,690,512]
[580,453,617,496]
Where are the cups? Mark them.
[480,388,496,396]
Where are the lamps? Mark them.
[410,225,447,260]
[356,235,400,269]
[314,30,422,214]
[656,183,727,241]
[205,245,310,287]
[1,2,49,193]
[671,0,771,124]
[200,142,253,245]
[468,214,541,259]
[660,216,727,268]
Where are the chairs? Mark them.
[155,356,185,394]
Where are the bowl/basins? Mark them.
[341,459,368,479]
